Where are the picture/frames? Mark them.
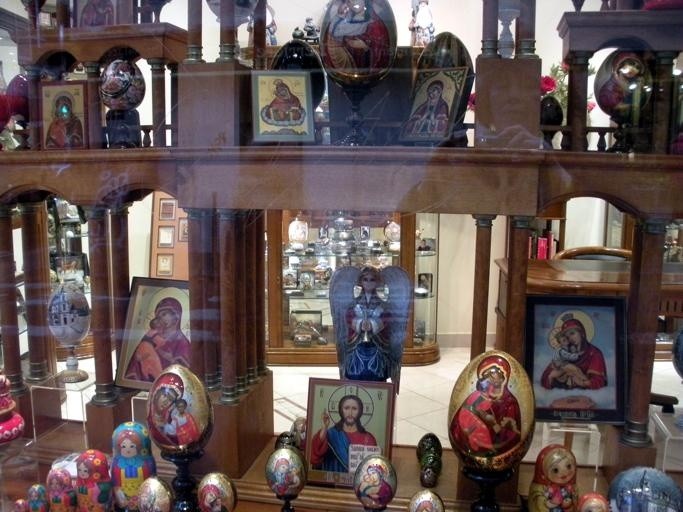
[251,70,315,142]
[156,198,189,276]
[304,377,396,489]
[524,294,628,425]
[114,277,189,390]
[398,66,469,141]
[37,80,89,149]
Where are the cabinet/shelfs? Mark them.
[265,209,441,367]
